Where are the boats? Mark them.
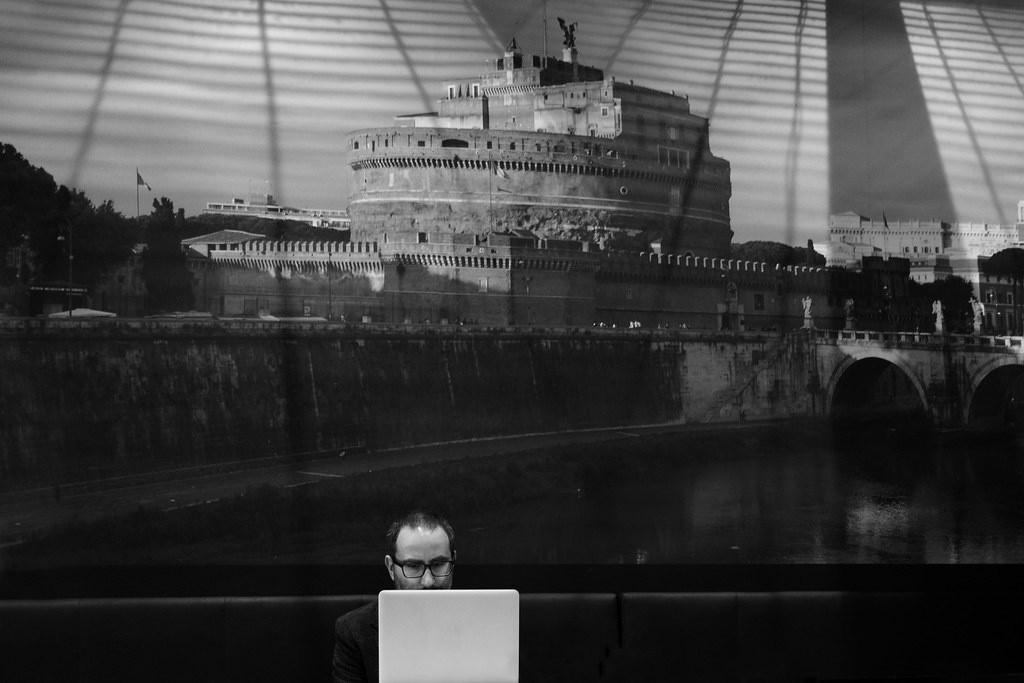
[176,10,843,332]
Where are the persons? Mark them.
[333,513,456,682]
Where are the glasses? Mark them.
[391,557,455,579]
